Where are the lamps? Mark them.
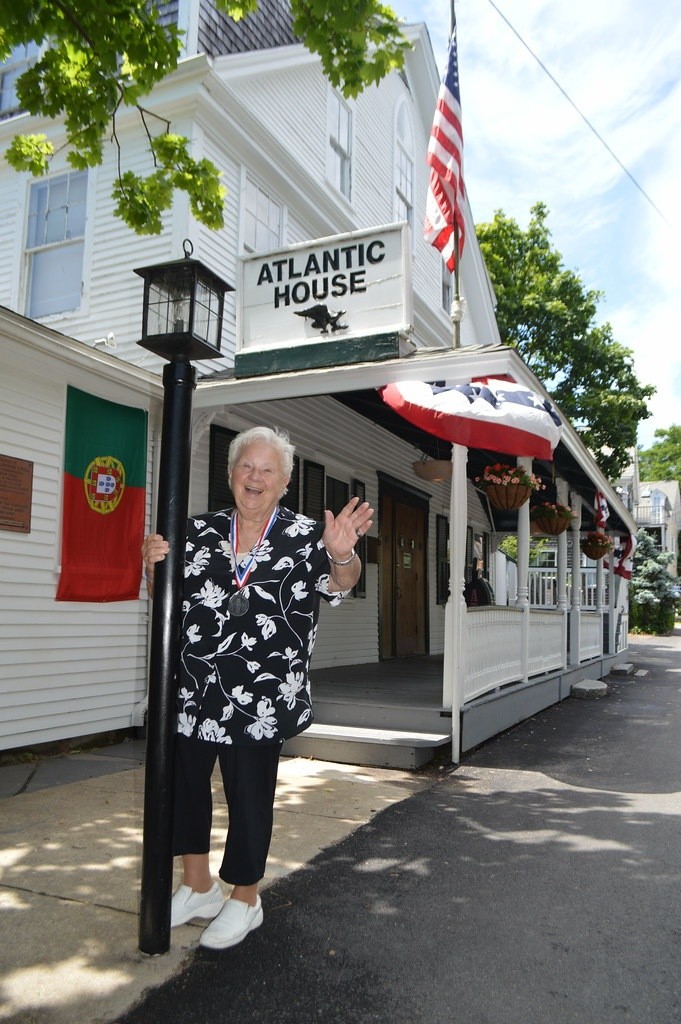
[412,439,452,482]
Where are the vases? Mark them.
[583,545,609,560]
[536,515,571,535]
[483,482,534,513]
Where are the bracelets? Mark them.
[326,547,356,565]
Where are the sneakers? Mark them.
[200,894,264,949]
[170,885,226,927]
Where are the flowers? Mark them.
[580,531,614,551]
[475,462,546,493]
[532,502,579,522]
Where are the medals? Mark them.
[228,595,249,617]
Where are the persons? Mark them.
[140,424,374,951]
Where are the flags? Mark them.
[422,22,465,276]
